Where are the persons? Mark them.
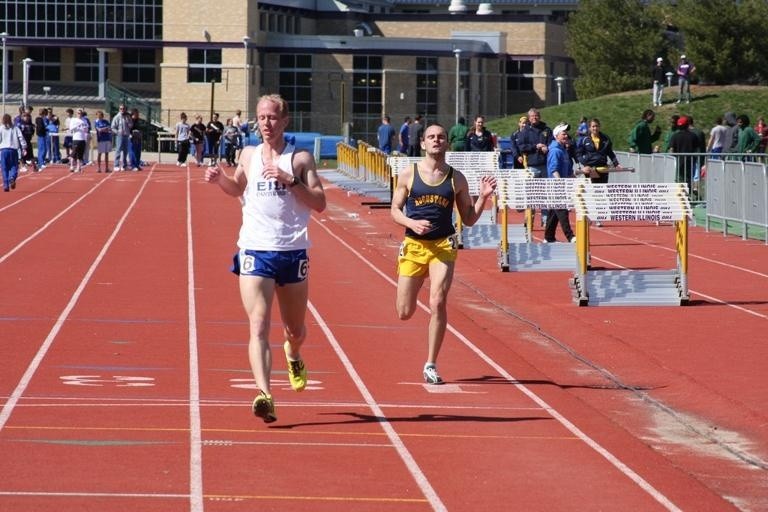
[204,95,326,422]
[377,113,425,157]
[174,110,244,168]
[652,57,667,107]
[448,117,469,151]
[512,107,622,245]
[663,112,768,205]
[390,123,496,385]
[0,102,143,191]
[627,110,661,155]
[464,115,495,151]
[676,54,696,104]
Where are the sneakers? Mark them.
[96,167,138,172]
[226,162,235,167]
[176,161,186,168]
[9,177,15,189]
[19,160,46,173]
[283,340,307,392]
[252,389,276,423]
[70,166,81,173]
[423,364,441,384]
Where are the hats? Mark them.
[678,117,687,126]
[553,124,570,137]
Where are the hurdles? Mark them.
[316,141,421,209]
[500,179,592,271]
[568,183,693,306]
[446,150,533,250]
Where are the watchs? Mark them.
[289,176,299,188]
